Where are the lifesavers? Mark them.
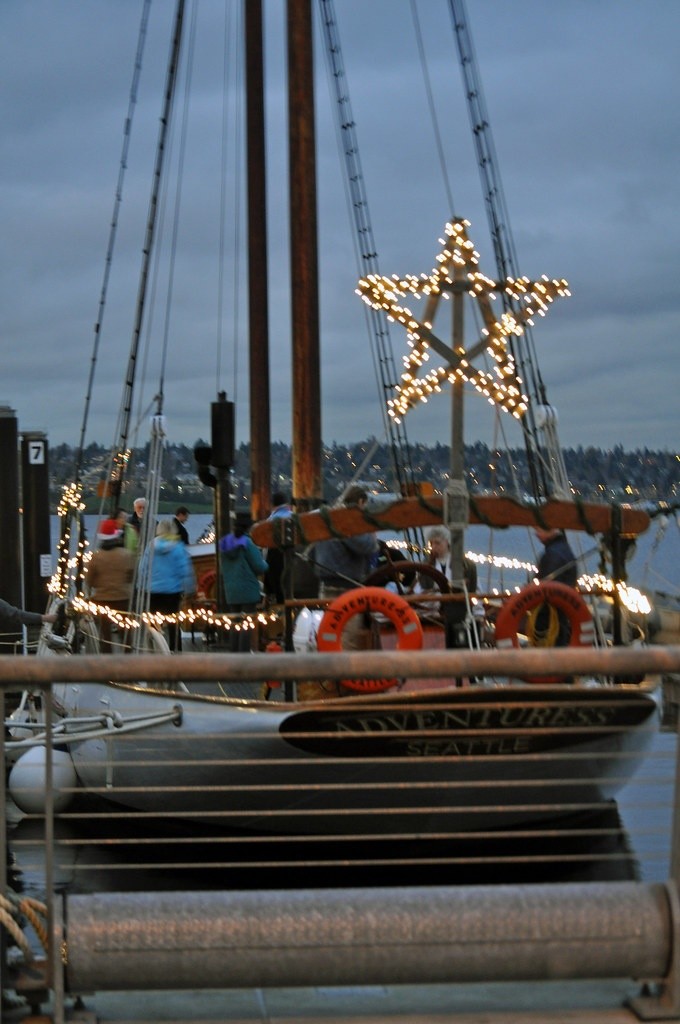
[492,579,596,686]
[315,585,424,694]
[197,571,220,613]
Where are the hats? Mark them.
[231,511,257,525]
[95,519,123,540]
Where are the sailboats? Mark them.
[1,0,680,847]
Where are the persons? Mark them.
[0,598,56,626]
[265,492,292,604]
[219,512,268,651]
[418,527,476,647]
[303,486,415,651]
[127,498,159,530]
[173,506,191,545]
[113,508,138,549]
[136,519,197,652]
[533,526,576,645]
[85,520,137,653]
